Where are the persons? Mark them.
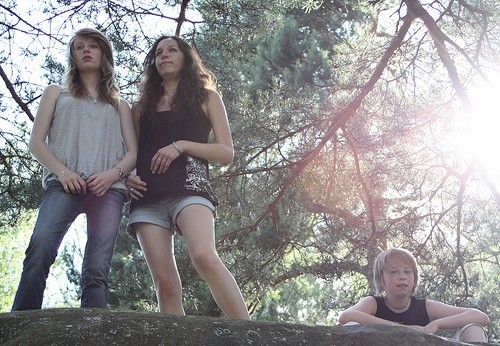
[125,36,250,321]
[339,248,490,343]
[11,28,138,312]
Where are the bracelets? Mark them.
[172,142,183,155]
[111,164,125,180]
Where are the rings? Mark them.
[131,188,133,191]
